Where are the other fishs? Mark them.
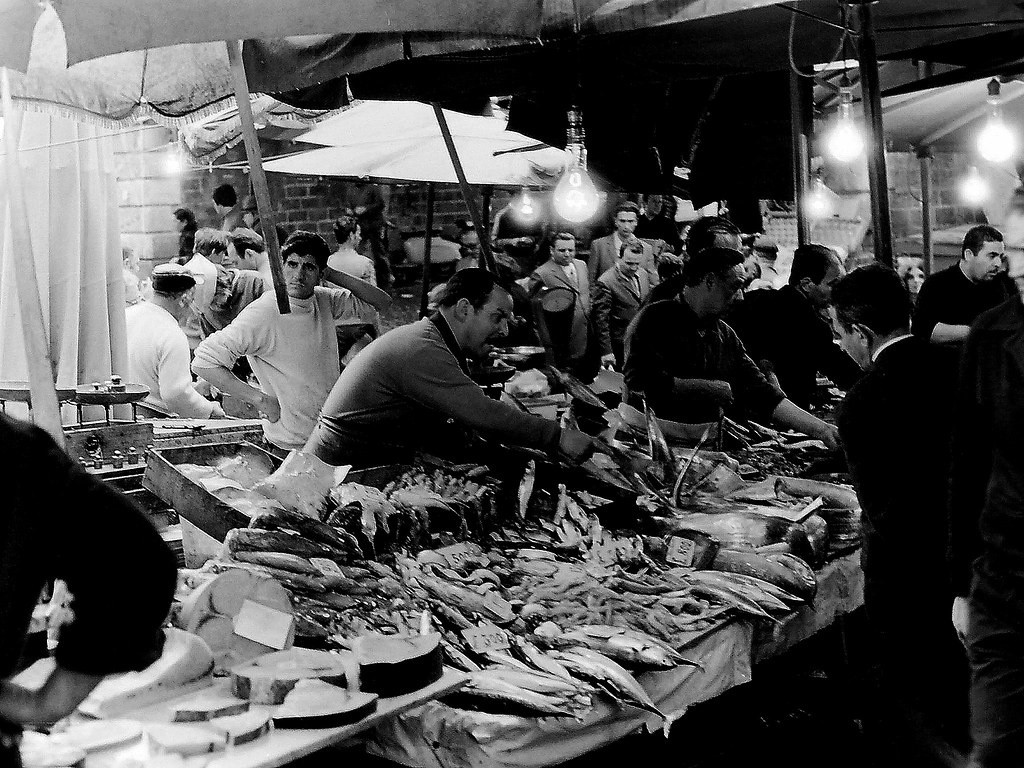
[185,454,822,739]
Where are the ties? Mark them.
[620,243,627,259]
[628,276,638,294]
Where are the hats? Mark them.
[152,263,196,299]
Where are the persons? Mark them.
[0,408,177,768]
[117,176,1024,563]
[953,275,1024,768]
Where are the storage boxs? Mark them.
[141,437,296,541]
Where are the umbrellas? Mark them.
[537,2,1022,265]
[261,101,574,325]
